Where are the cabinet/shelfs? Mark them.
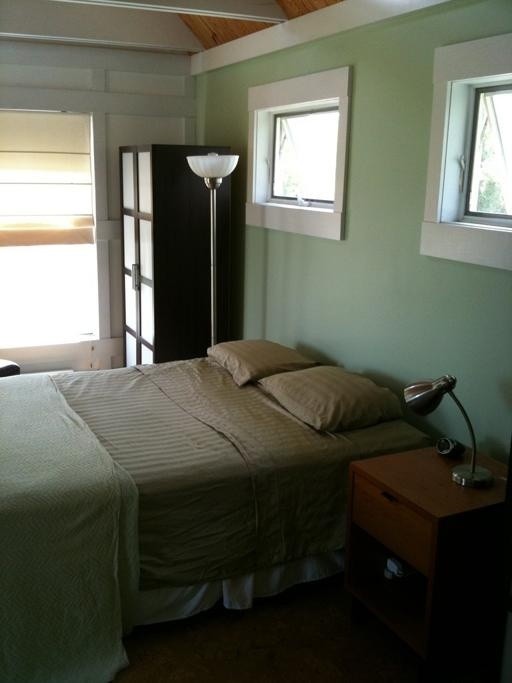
[116,140,245,369]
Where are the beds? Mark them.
[1,355,433,681]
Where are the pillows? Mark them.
[254,360,406,436]
[206,336,323,388]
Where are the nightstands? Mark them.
[338,437,512,674]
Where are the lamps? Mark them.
[179,148,242,351]
[401,370,496,490]
